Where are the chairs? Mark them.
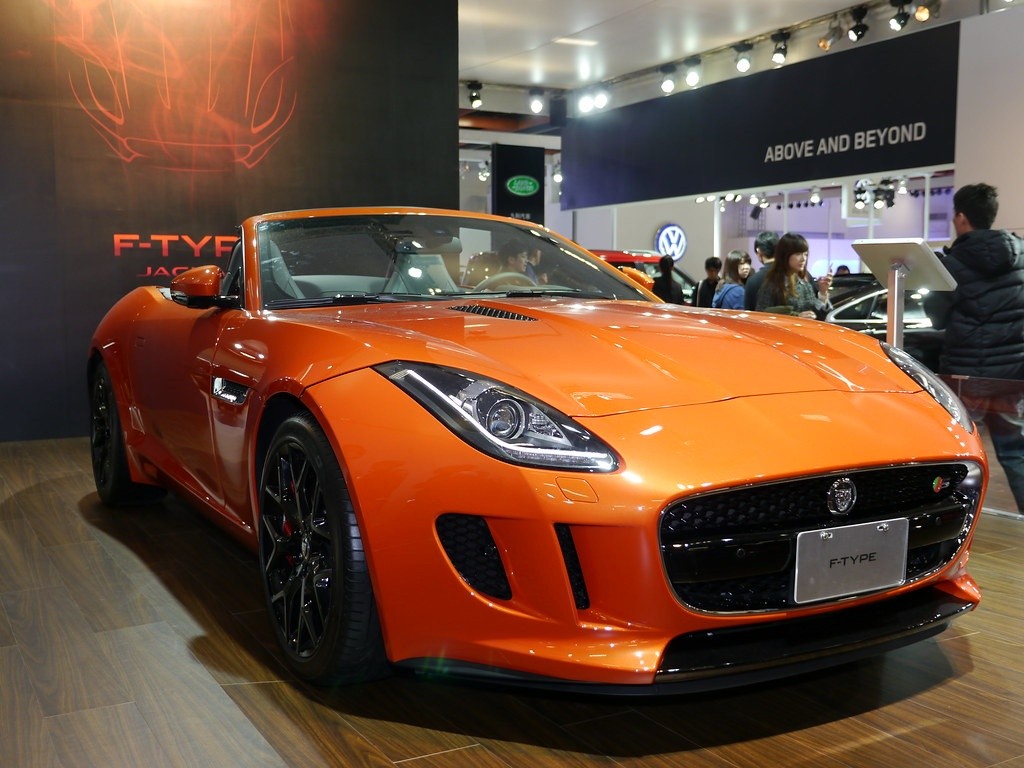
[381,238,459,295]
[220,237,305,303]
[460,251,500,287]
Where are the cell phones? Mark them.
[828,262,833,273]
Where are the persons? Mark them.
[923,184,1024,515]
[651,254,684,305]
[483,236,548,292]
[691,231,852,321]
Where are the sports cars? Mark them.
[78,204,990,704]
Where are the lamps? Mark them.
[460,161,471,180]
[847,4,870,43]
[732,43,753,73]
[797,201,802,207]
[854,175,951,210]
[478,166,491,181]
[552,162,562,183]
[777,202,782,209]
[467,80,483,109]
[720,192,771,219]
[771,32,791,64]
[804,201,808,207]
[811,187,824,207]
[789,201,794,208]
[889,0,913,32]
[914,0,941,22]
[683,58,702,87]
[529,87,544,114]
[818,14,844,52]
[660,65,678,93]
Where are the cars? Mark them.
[588,248,697,307]
[823,272,947,378]
